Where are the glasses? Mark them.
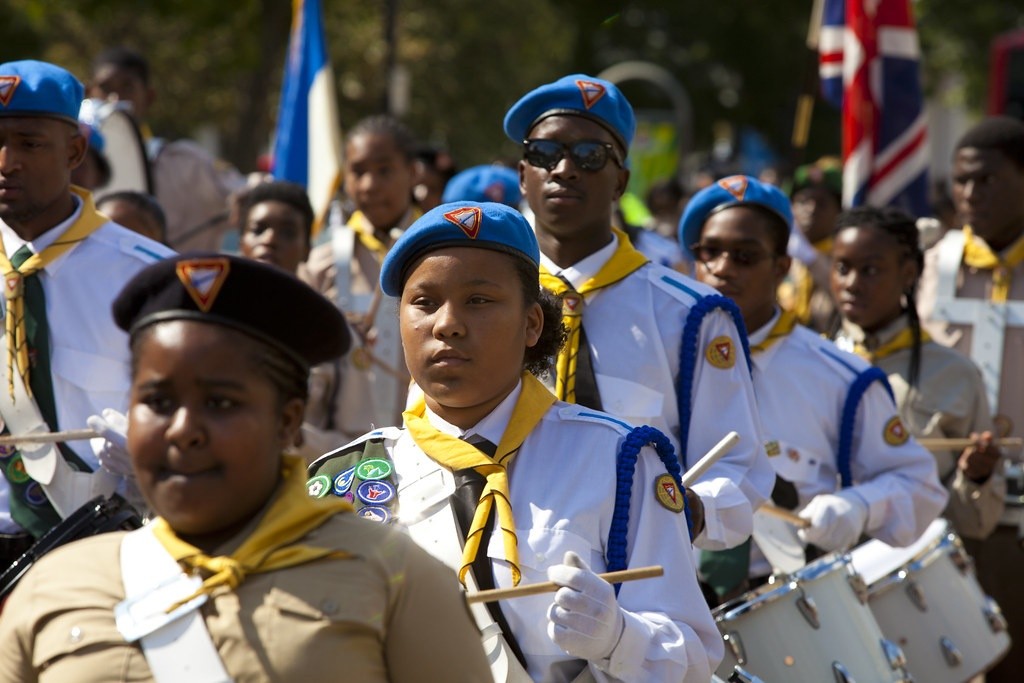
[522,139,621,172]
[691,242,774,266]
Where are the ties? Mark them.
[402,369,559,591]
[748,306,797,355]
[855,327,932,364]
[351,208,418,267]
[550,275,603,412]
[963,225,1024,301]
[1,183,112,405]
[152,449,355,614]
[1,242,96,542]
[448,440,529,669]
[536,224,652,404]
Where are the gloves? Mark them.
[546,551,625,662]
[796,489,868,551]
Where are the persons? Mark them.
[0,252,498,683]
[74,58,533,436]
[305,200,726,683]
[0,59,187,574]
[399,75,778,550]
[618,69,1024,683]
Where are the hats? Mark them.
[679,175,792,259]
[0,60,85,127]
[504,73,635,155]
[78,125,112,185]
[380,200,539,299]
[112,251,353,376]
[444,163,523,210]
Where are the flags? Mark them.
[818,0,933,220]
[271,1,350,239]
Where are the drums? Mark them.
[1,489,144,609]
[848,514,1015,683]
[710,544,912,682]
